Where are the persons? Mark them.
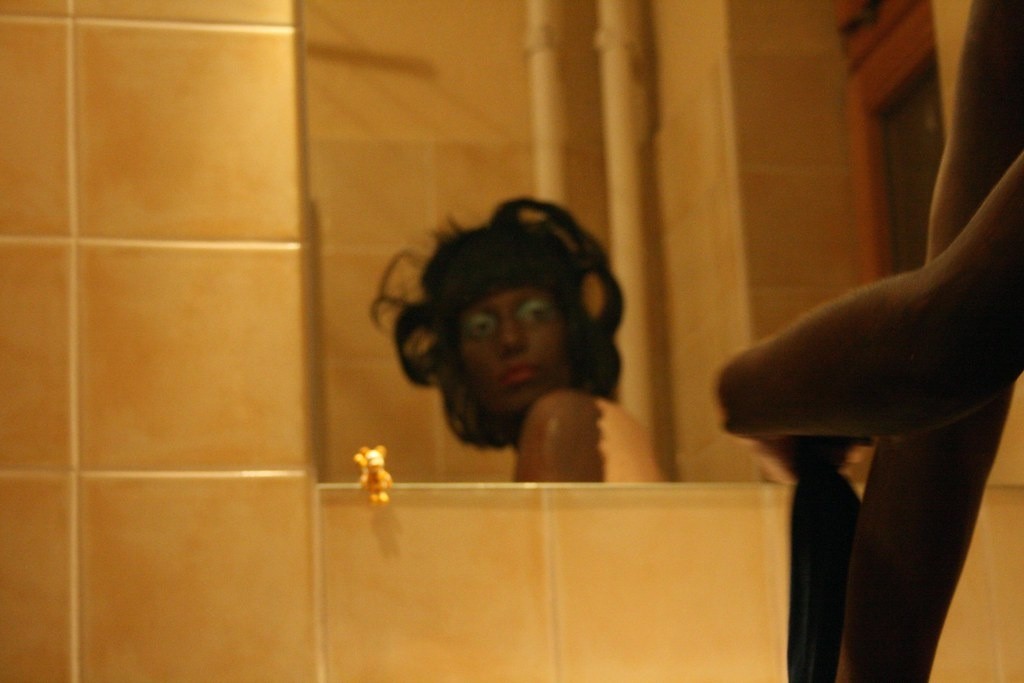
[712,0,1024,683]
[370,195,666,482]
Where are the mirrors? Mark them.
[294,1,978,484]
